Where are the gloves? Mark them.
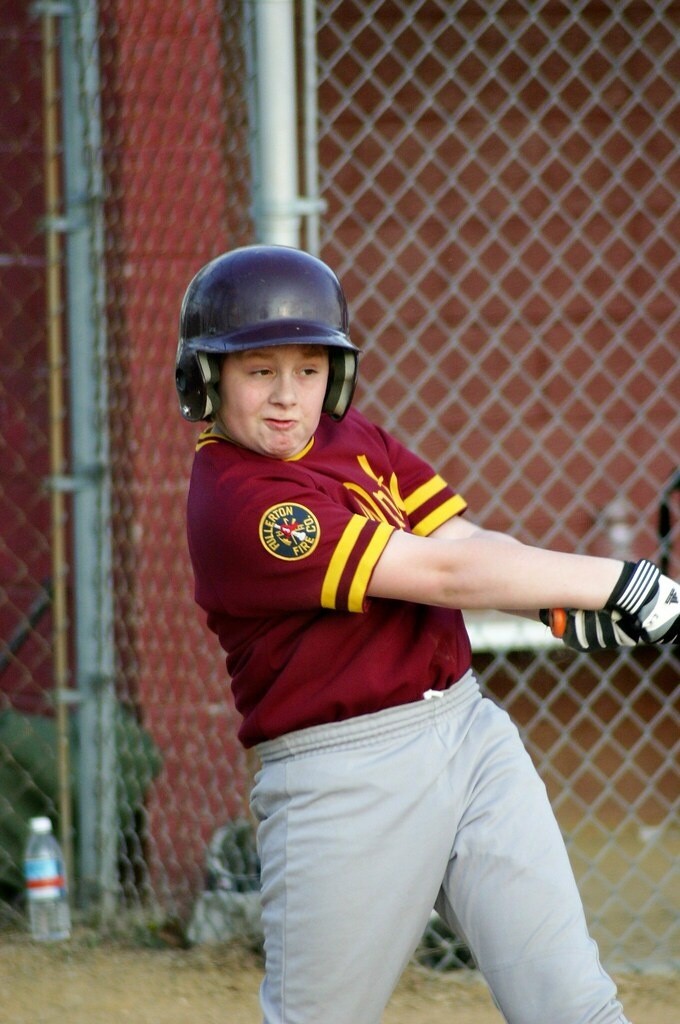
[552,608,639,652]
[618,561,680,643]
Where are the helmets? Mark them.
[175,244,363,423]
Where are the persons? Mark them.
[173,245,680,1024]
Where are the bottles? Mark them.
[24,815,69,945]
